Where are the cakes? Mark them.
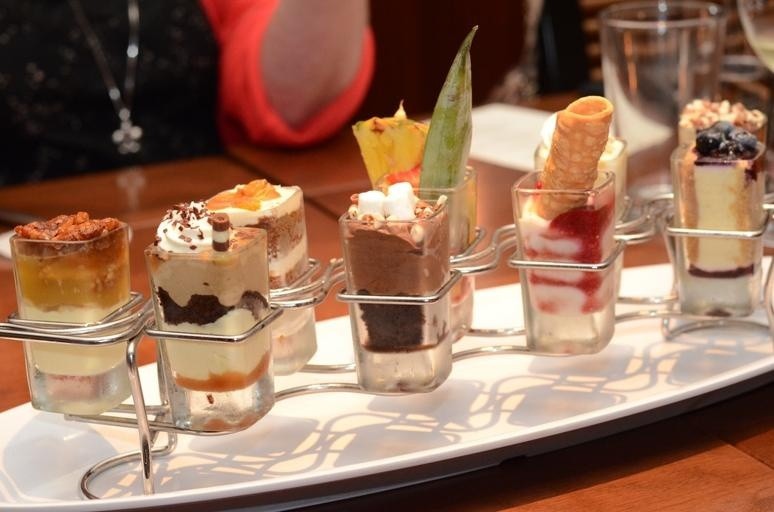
[204,178,315,340]
[342,181,452,354]
[146,199,271,391]
[12,210,132,378]
[351,100,475,309]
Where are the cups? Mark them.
[142,220,271,436]
[599,2,727,204]
[512,135,631,354]
[12,218,136,417]
[197,181,320,376]
[339,197,454,394]
[370,156,478,343]
[670,110,768,318]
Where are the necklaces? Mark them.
[68,0,146,158]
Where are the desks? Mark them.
[0,91,774,512]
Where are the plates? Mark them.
[0,255,774,512]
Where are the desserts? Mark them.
[676,120,765,304]
[512,95,616,342]
[535,110,626,270]
[677,96,767,169]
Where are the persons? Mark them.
[0,0,373,188]
